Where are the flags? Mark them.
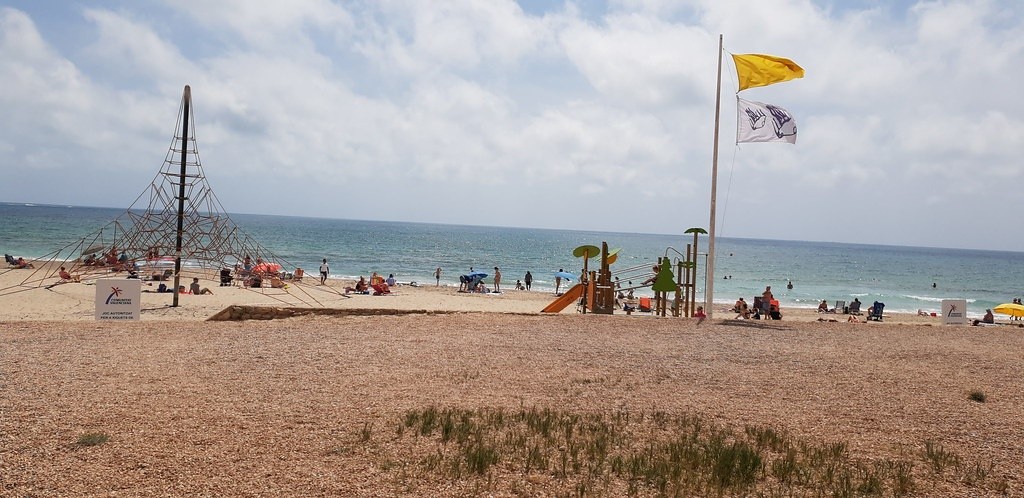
[735,96,797,144]
[731,53,805,93]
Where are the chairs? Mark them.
[293,268,304,281]
[850,301,861,313]
[5,254,20,268]
[370,275,378,284]
[834,301,845,313]
[871,302,885,320]
[58,274,80,283]
[371,284,390,296]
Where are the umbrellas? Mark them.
[465,271,488,289]
[251,262,281,276]
[550,271,579,291]
[81,244,106,261]
[993,303,1024,321]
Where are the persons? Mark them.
[355,273,397,295]
[732,286,783,320]
[59,267,80,280]
[818,300,828,312]
[319,258,329,285]
[84,246,214,295]
[849,298,861,314]
[221,254,287,288]
[433,266,563,293]
[18,256,35,269]
[1009,297,1023,321]
[848,315,867,323]
[579,265,706,317]
[723,275,727,279]
[983,308,994,323]
[867,301,879,320]
[787,281,793,289]
[933,283,936,288]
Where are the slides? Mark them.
[541,283,584,312]
